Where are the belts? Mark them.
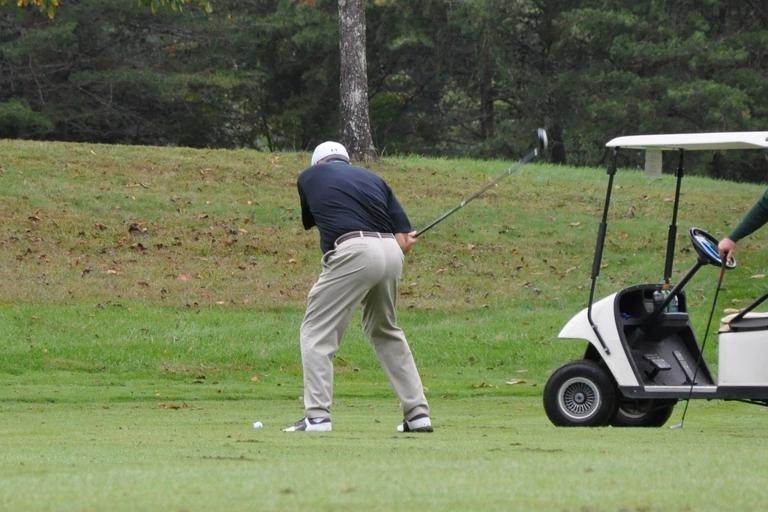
[337,231,393,245]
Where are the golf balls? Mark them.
[254,422,262,429]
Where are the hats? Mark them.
[311,141,349,166]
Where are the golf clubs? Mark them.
[667,251,728,430]
[415,128,547,238]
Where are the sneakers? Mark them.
[282,417,331,432]
[397,414,433,431]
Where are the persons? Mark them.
[280,140,436,434]
[716,188,768,266]
